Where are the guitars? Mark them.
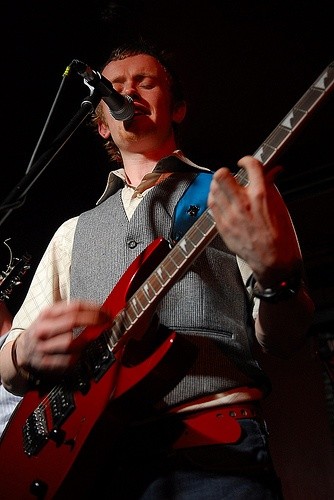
[0,53,334,500]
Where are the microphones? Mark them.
[71,60,134,121]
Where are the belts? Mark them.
[161,386,260,448]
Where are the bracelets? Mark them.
[9,327,44,383]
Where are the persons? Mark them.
[0,38,316,500]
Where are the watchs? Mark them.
[248,262,305,307]
